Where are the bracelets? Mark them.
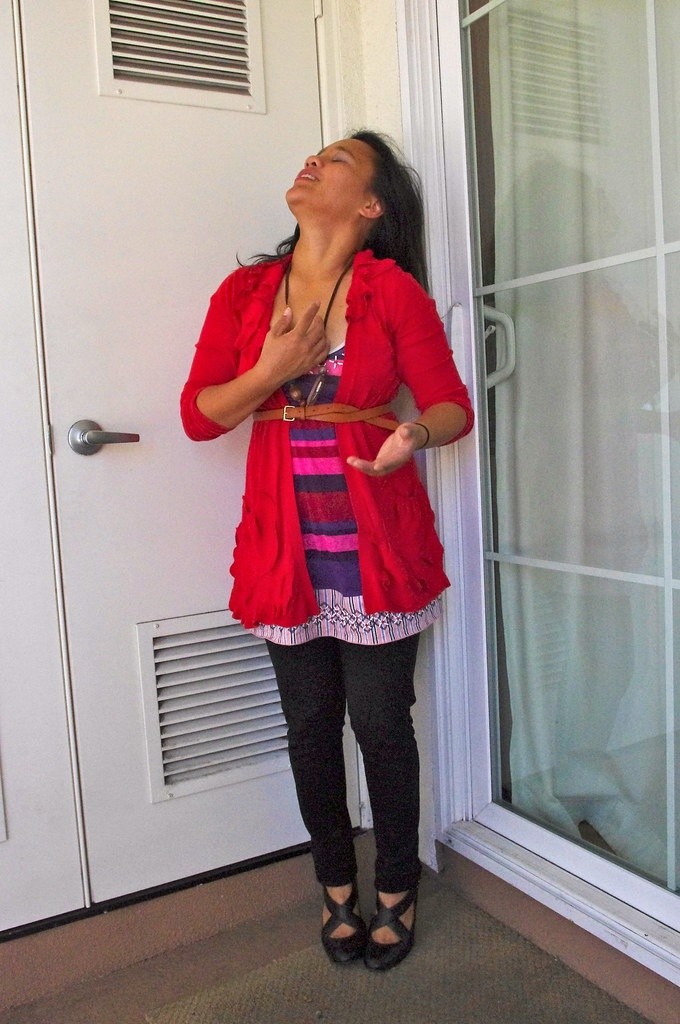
[414,423,430,450]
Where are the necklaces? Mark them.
[284,250,356,409]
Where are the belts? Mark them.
[253,402,400,432]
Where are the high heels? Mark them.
[363,882,417,970]
[321,877,367,964]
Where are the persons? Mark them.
[180,130,477,973]
